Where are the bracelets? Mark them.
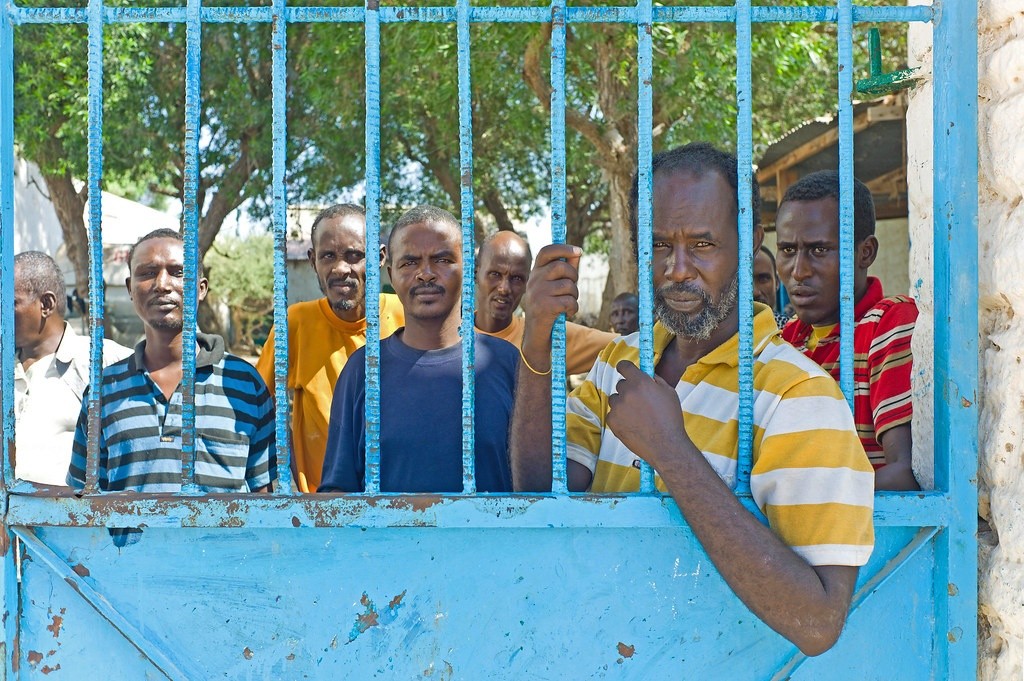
[519,339,551,375]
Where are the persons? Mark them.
[609,293,639,335]
[14,251,136,486]
[774,169,921,491]
[474,231,618,376]
[753,246,790,330]
[509,144,875,658]
[66,227,277,493]
[316,205,521,493]
[254,205,405,493]
